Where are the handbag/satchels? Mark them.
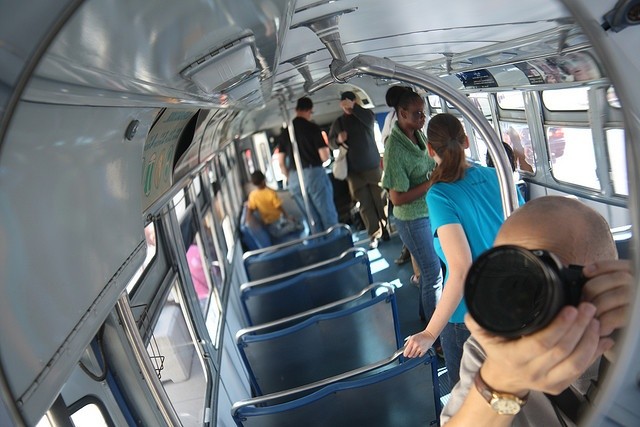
[333,149,347,180]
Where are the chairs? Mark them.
[237,282,396,400]
[239,246,376,334]
[237,206,310,249]
[240,221,356,282]
[212,345,438,427]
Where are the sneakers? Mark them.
[369,238,379,249]
[382,232,389,240]
[410,275,420,288]
[394,252,410,264]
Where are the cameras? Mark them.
[462,243,585,337]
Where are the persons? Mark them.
[404,112,526,388]
[244,170,305,240]
[440,196,640,426]
[329,91,391,248]
[382,86,445,358]
[279,97,341,243]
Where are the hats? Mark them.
[342,92,356,100]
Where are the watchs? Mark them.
[473,367,528,417]
